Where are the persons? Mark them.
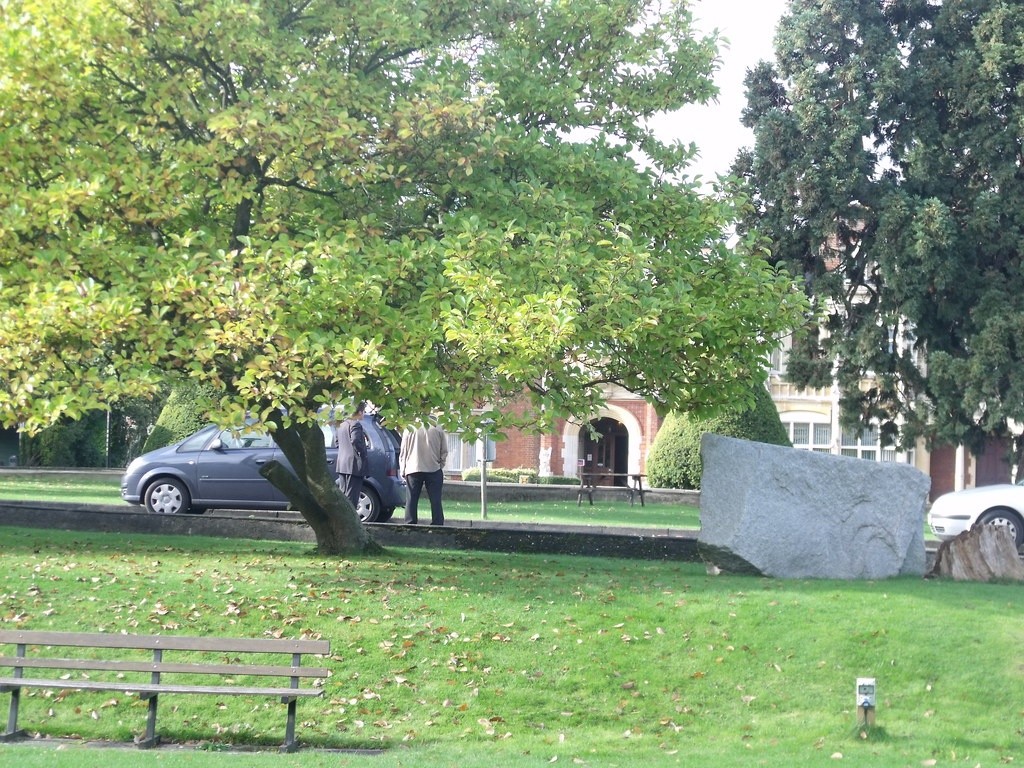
[398,406,448,526]
[335,400,371,513]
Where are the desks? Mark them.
[576,472,645,507]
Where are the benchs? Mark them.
[572,488,653,493]
[0,629,330,753]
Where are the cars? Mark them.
[120,407,408,522]
[926,477,1024,551]
